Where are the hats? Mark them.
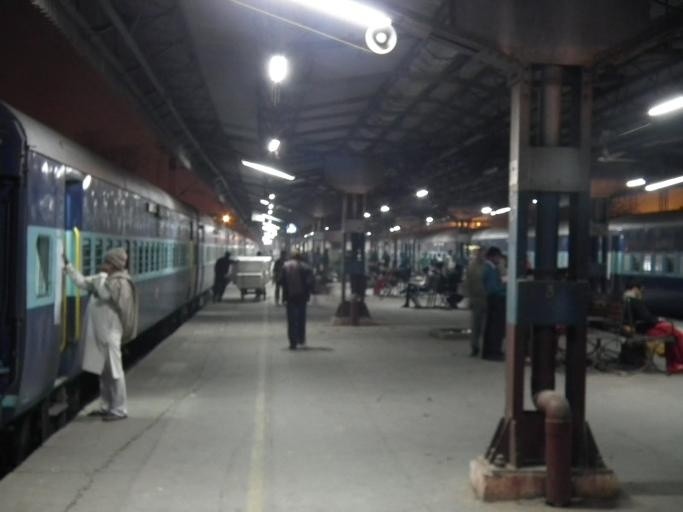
[105,248,128,269]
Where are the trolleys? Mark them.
[240,287,266,303]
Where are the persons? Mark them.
[64,242,133,421]
[623,282,683,375]
[211,245,505,363]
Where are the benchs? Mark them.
[407,274,460,308]
[584,295,676,379]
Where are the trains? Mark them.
[364,212,682,317]
[0,99,266,474]
